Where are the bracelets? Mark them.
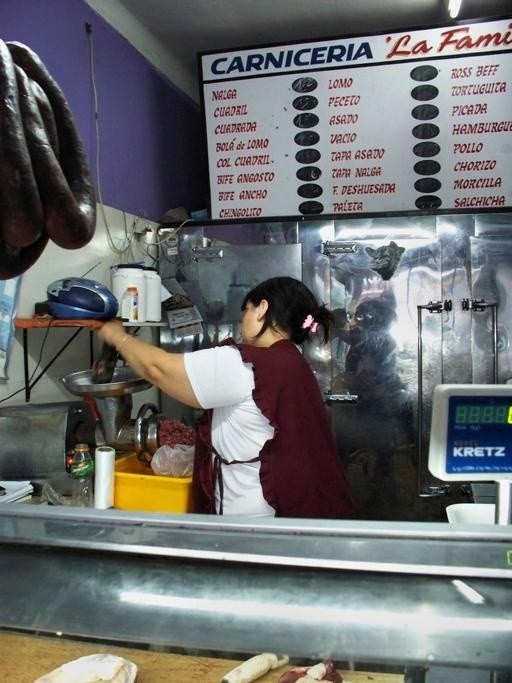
[115,334,130,353]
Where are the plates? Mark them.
[32,655,138,683]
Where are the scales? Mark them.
[427,383,512,525]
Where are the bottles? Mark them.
[70,443,93,510]
[110,263,162,326]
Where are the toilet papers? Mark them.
[93,447,114,510]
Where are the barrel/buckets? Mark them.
[108,264,145,321]
[145,264,163,323]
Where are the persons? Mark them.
[331,289,402,395]
[96,275,362,519]
